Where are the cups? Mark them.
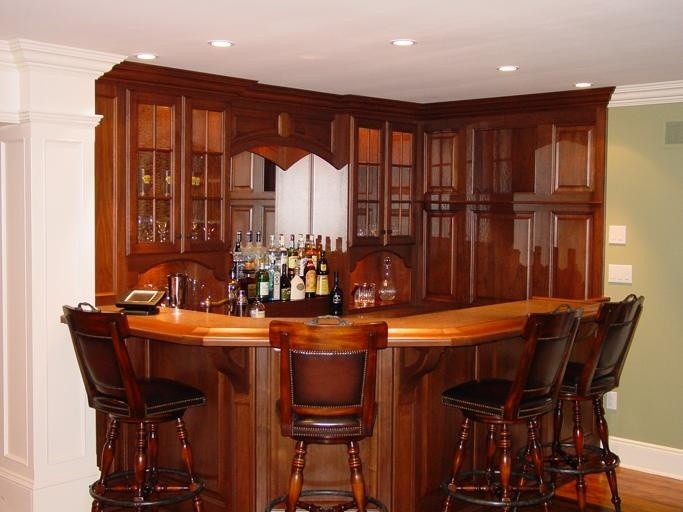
[353,281,376,307]
[187,281,203,312]
[160,168,170,197]
[166,272,189,309]
[138,215,216,243]
[138,166,152,197]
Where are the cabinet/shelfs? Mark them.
[94,60,616,320]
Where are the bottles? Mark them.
[377,256,396,301]
[191,168,202,194]
[226,231,330,317]
[328,271,343,317]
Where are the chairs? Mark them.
[438,304,582,512]
[61,304,206,511]
[524,294,646,512]
[267,319,389,512]
